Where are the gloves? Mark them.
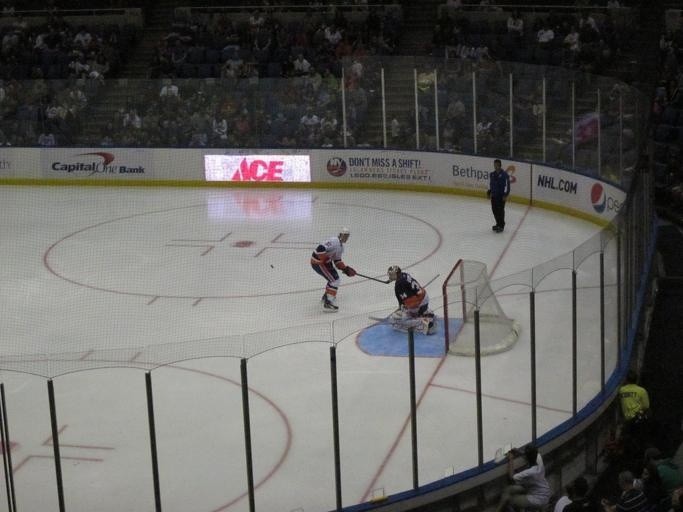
[342,266,356,277]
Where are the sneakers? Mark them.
[324,298,339,309]
[321,293,326,300]
[422,318,430,336]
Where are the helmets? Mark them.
[387,265,401,280]
[338,227,350,243]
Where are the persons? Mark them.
[554,418,683,512]
[386,265,439,337]
[486,158,511,233]
[654,28,681,218]
[618,368,657,476]
[495,442,552,511]
[1,1,151,148]
[309,227,357,313]
[391,1,642,182]
[98,1,399,150]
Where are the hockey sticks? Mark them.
[342,271,392,284]
[369,274,440,322]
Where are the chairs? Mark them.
[517,194,683,512]
